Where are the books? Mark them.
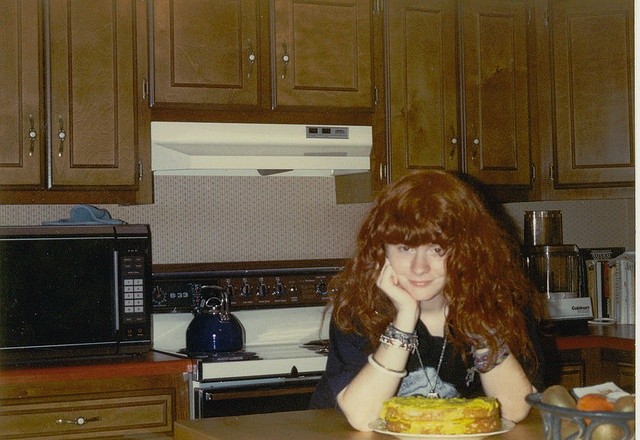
[578,246,636,325]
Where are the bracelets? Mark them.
[467,322,511,373]
[378,334,414,351]
[383,324,419,344]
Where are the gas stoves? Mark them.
[153,266,336,383]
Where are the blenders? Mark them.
[520,211,594,332]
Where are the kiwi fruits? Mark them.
[542,384,583,437]
[612,395,635,435]
[590,424,626,440]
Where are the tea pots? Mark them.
[186,286,244,353]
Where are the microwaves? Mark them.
[0,224,153,367]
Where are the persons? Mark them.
[309,170,546,434]
[367,353,407,378]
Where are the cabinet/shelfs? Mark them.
[541,1,639,199]
[545,323,636,390]
[0,359,189,439]
[0,0,150,201]
[152,0,378,123]
[381,0,539,202]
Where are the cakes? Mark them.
[382,394,502,436]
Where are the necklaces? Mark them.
[413,332,449,398]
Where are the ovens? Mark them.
[194,379,322,421]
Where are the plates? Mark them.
[369,417,516,440]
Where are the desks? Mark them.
[172,393,635,440]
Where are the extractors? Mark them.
[150,122,374,178]
